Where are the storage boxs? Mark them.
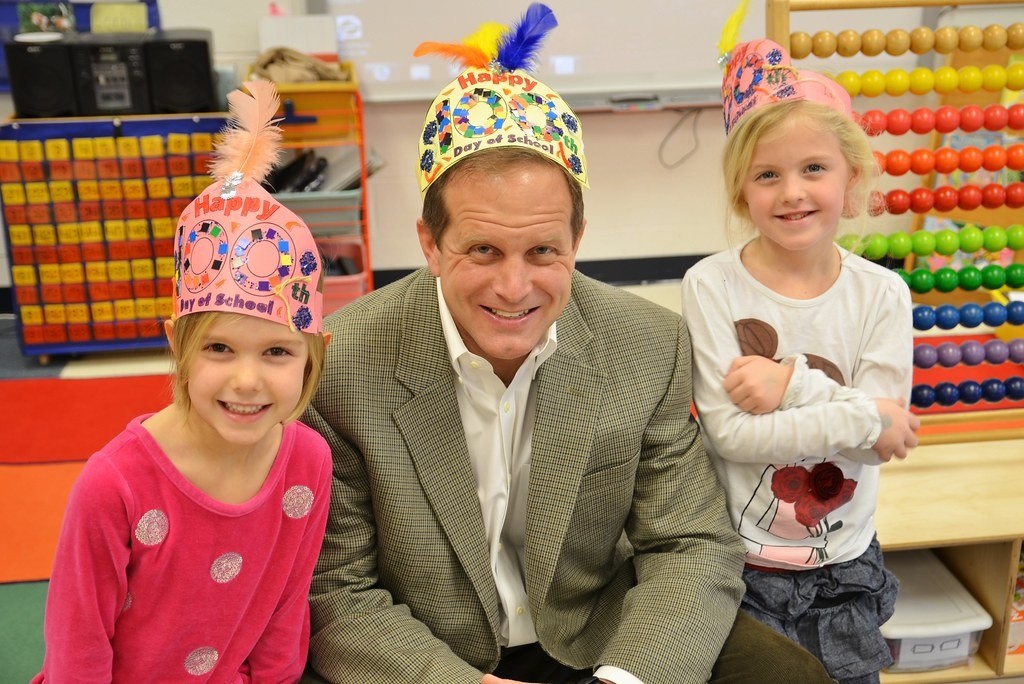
[877,554,996,672]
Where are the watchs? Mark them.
[585,677,607,684]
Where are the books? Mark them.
[267,143,384,194]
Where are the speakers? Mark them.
[144,30,219,113]
[5,32,95,117]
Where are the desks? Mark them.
[0,112,248,360]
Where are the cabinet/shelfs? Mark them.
[874,439,1024,684]
[243,55,374,317]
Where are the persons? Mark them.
[31,260,332,684]
[295,146,840,684]
[682,98,921,684]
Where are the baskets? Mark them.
[270,188,360,236]
[316,238,367,317]
[242,61,357,140]
